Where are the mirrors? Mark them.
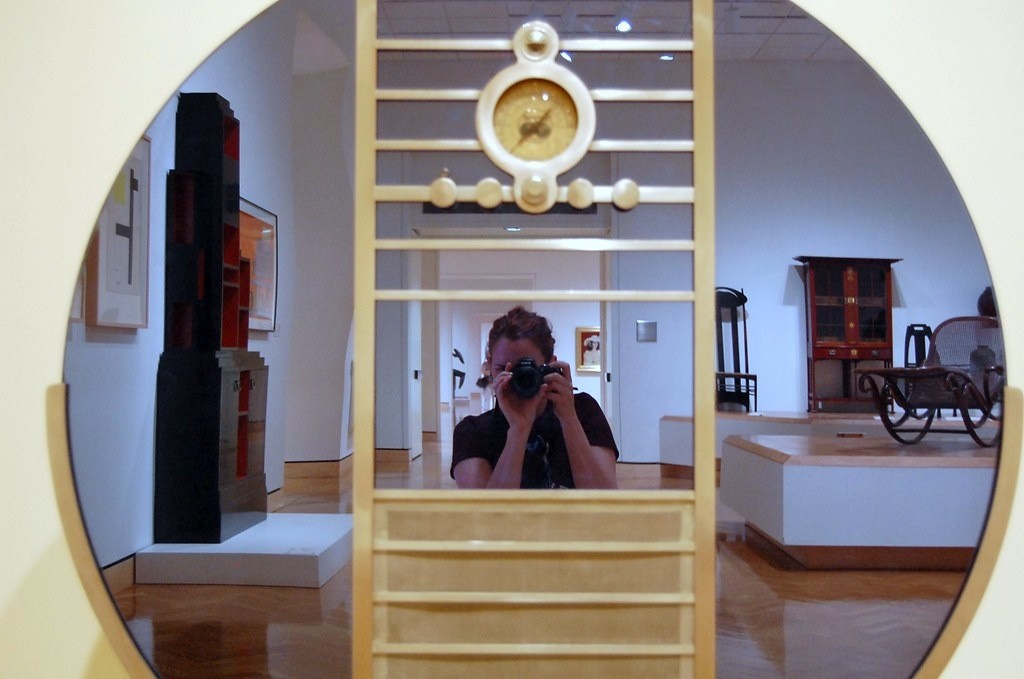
[44,0,1008,679]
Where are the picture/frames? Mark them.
[85,135,151,328]
[575,326,601,372]
[239,195,279,332]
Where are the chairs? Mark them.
[853,315,1004,447]
[715,286,757,412]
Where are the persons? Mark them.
[582,335,601,366]
[451,306,620,491]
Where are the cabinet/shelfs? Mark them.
[792,255,904,414]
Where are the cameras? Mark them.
[509,357,563,399]
